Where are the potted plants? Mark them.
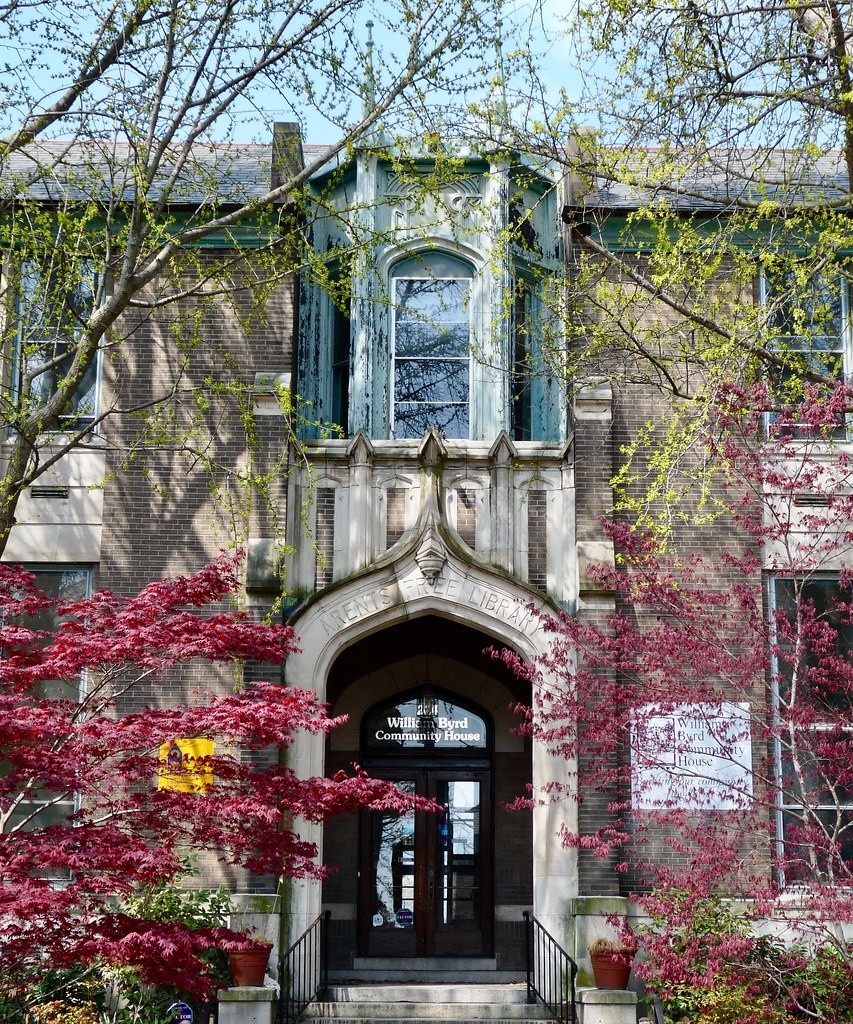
[587,938,638,990]
[227,933,275,987]
